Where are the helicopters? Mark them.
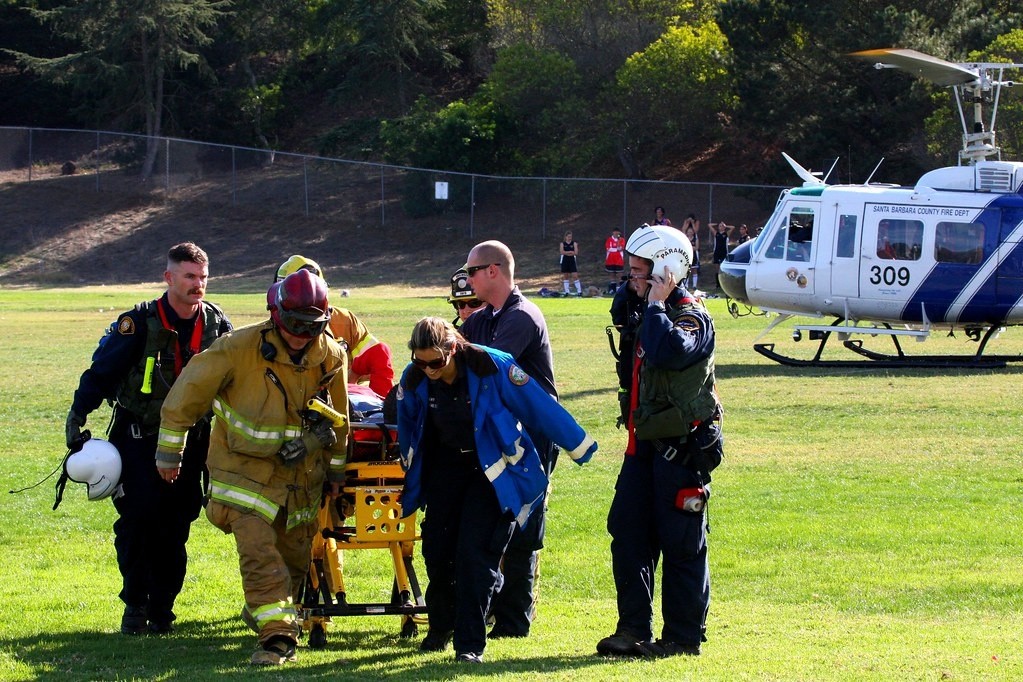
[718,49,1023,369]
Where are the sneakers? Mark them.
[636,636,701,657]
[456,649,484,664]
[597,631,645,655]
[420,624,454,653]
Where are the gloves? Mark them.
[65,415,91,449]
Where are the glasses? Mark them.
[411,350,450,369]
[456,298,486,309]
[467,263,501,277]
[284,311,331,337]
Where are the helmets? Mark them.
[64,437,124,501]
[447,263,479,302]
[625,222,694,285]
[266,268,329,339]
[277,254,323,281]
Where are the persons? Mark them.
[277,255,396,602]
[66,241,234,636]
[605,227,625,294]
[559,230,583,298]
[447,240,559,637]
[395,316,598,663]
[650,207,764,288]
[596,225,724,659]
[154,269,350,665]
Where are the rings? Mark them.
[657,280,663,283]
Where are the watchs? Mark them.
[648,299,666,311]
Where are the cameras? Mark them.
[278,425,338,464]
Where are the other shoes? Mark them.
[487,625,513,638]
[252,637,296,666]
[148,620,173,635]
[120,608,146,634]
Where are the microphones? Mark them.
[621,274,646,281]
